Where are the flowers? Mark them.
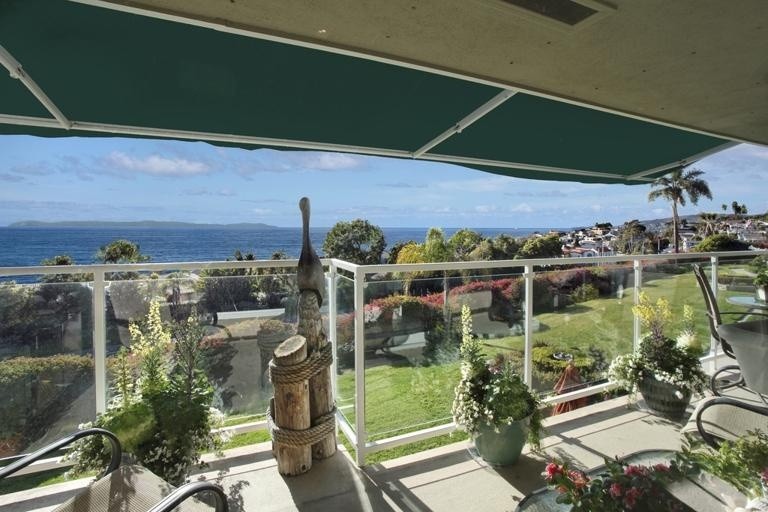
[63,294,232,478]
[442,303,546,438]
[602,292,712,398]
[545,456,680,512]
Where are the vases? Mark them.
[468,418,533,468]
[146,456,188,489]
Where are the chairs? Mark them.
[684,259,767,398]
[1,426,236,512]
[667,394,766,455]
[716,314,767,403]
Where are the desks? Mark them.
[508,448,767,512]
[718,295,767,322]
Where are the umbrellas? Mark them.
[554,359,586,416]
